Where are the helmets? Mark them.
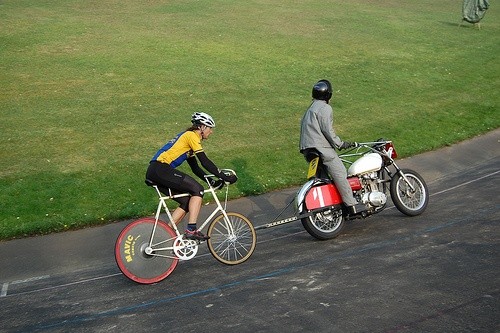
[312,80,332,100]
[191,112,215,127]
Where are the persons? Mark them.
[146,112,237,243]
[298,79,373,215]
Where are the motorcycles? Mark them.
[294,138,429,240]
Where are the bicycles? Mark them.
[114,169,257,284]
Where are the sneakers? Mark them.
[184,229,210,240]
[351,204,366,214]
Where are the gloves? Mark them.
[213,180,224,190]
[341,142,351,149]
[225,176,237,184]
